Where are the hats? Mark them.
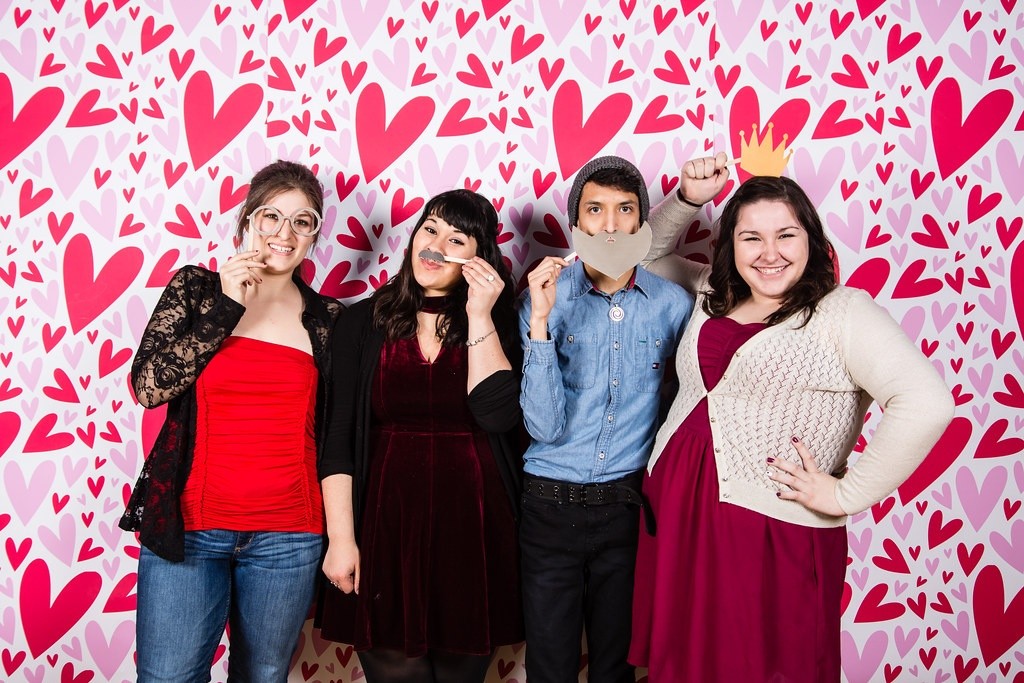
[568,155,650,233]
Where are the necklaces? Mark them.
[604,292,626,321]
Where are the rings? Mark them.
[488,275,495,282]
[330,581,340,589]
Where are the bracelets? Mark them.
[466,329,497,347]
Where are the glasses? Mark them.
[246,205,323,237]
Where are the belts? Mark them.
[519,474,658,537]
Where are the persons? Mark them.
[626,153,955,683]
[315,189,530,683]
[120,161,348,683]
[514,155,695,683]
